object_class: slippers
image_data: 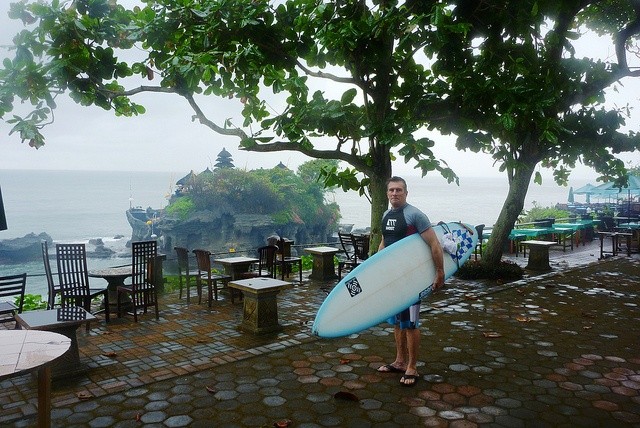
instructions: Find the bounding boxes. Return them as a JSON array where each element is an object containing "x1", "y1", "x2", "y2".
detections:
[
  {"x1": 376, "y1": 363, "x2": 406, "y2": 373},
  {"x1": 400, "y1": 370, "x2": 420, "y2": 387}
]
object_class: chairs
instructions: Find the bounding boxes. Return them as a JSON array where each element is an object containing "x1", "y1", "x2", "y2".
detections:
[
  {"x1": 337, "y1": 231, "x2": 369, "y2": 260},
  {"x1": 273, "y1": 242, "x2": 303, "y2": 282},
  {"x1": 41, "y1": 239, "x2": 87, "y2": 310},
  {"x1": 192, "y1": 247, "x2": 235, "y2": 308},
  {"x1": 244, "y1": 245, "x2": 279, "y2": 279},
  {"x1": 0, "y1": 272, "x2": 27, "y2": 330},
  {"x1": 174, "y1": 245, "x2": 207, "y2": 304},
  {"x1": 116, "y1": 240, "x2": 160, "y2": 324},
  {"x1": 468, "y1": 224, "x2": 486, "y2": 261},
  {"x1": 55, "y1": 242, "x2": 111, "y2": 332}
]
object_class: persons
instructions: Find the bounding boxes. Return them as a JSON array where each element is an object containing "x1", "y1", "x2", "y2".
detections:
[{"x1": 376, "y1": 176, "x2": 445, "y2": 388}]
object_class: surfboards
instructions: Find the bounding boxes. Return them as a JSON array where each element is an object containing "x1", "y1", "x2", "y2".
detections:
[{"x1": 310, "y1": 222, "x2": 479, "y2": 339}]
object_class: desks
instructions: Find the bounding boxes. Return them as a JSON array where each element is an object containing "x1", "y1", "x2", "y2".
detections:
[
  {"x1": 520, "y1": 239, "x2": 558, "y2": 271},
  {"x1": 482, "y1": 233, "x2": 527, "y2": 254},
  {"x1": 88, "y1": 268, "x2": 146, "y2": 310},
  {"x1": 213, "y1": 256, "x2": 260, "y2": 296},
  {"x1": 597, "y1": 231, "x2": 634, "y2": 261},
  {"x1": 227, "y1": 276, "x2": 294, "y2": 334},
  {"x1": 0, "y1": 327, "x2": 72, "y2": 428},
  {"x1": 556, "y1": 222, "x2": 586, "y2": 248},
  {"x1": 548, "y1": 227, "x2": 574, "y2": 253},
  {"x1": 14, "y1": 306, "x2": 97, "y2": 380},
  {"x1": 577, "y1": 219, "x2": 602, "y2": 242},
  {"x1": 569, "y1": 204, "x2": 639, "y2": 220},
  {"x1": 620, "y1": 222, "x2": 640, "y2": 248},
  {"x1": 512, "y1": 227, "x2": 550, "y2": 252},
  {"x1": 304, "y1": 244, "x2": 340, "y2": 281}
]
